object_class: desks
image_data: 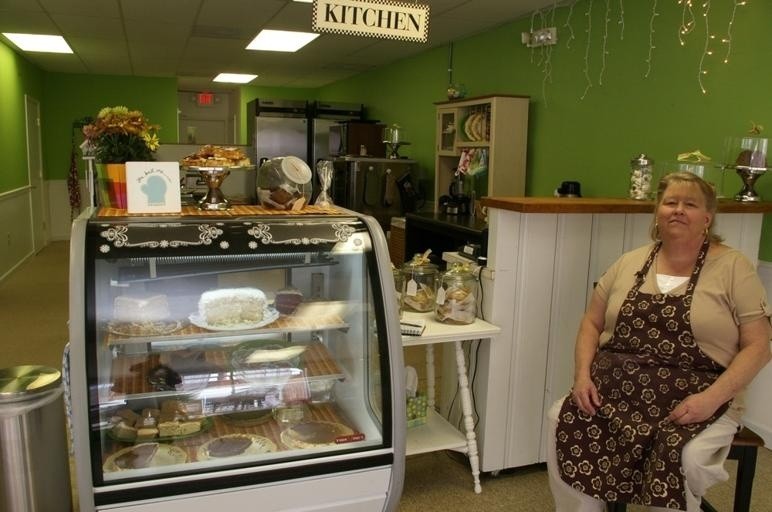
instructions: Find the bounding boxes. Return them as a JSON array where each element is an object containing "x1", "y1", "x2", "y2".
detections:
[{"x1": 371, "y1": 310, "x2": 502, "y2": 495}]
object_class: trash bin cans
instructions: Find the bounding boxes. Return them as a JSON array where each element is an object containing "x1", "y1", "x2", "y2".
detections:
[{"x1": 1, "y1": 365, "x2": 72, "y2": 511}]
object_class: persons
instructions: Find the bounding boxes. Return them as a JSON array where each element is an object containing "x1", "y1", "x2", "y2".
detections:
[{"x1": 544, "y1": 170, "x2": 772, "y2": 512}]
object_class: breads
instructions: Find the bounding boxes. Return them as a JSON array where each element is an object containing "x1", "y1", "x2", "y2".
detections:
[
  {"x1": 109, "y1": 400, "x2": 202, "y2": 440},
  {"x1": 181, "y1": 143, "x2": 250, "y2": 167}
]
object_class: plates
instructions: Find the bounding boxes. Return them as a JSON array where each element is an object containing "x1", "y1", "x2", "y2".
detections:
[{"x1": 465, "y1": 114, "x2": 489, "y2": 141}]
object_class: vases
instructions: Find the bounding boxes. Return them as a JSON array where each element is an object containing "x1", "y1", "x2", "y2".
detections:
[{"x1": 96, "y1": 165, "x2": 126, "y2": 209}]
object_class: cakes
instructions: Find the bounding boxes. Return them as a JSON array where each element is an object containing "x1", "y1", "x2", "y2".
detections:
[
  {"x1": 114, "y1": 444, "x2": 158, "y2": 468},
  {"x1": 293, "y1": 423, "x2": 342, "y2": 443},
  {"x1": 200, "y1": 288, "x2": 265, "y2": 323},
  {"x1": 207, "y1": 436, "x2": 252, "y2": 457},
  {"x1": 112, "y1": 292, "x2": 170, "y2": 320},
  {"x1": 275, "y1": 287, "x2": 303, "y2": 315}
]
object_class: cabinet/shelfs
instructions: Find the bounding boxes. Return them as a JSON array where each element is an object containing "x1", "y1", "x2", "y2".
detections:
[
  {"x1": 403, "y1": 211, "x2": 488, "y2": 273},
  {"x1": 70, "y1": 205, "x2": 406, "y2": 512},
  {"x1": 434, "y1": 94, "x2": 533, "y2": 215},
  {"x1": 330, "y1": 155, "x2": 417, "y2": 232},
  {"x1": 440, "y1": 193, "x2": 771, "y2": 477}
]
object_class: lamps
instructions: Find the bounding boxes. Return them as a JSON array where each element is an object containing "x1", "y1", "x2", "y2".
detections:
[{"x1": 520, "y1": 27, "x2": 557, "y2": 48}]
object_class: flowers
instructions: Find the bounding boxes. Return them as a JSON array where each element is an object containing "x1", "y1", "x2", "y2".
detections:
[{"x1": 81, "y1": 104, "x2": 162, "y2": 162}]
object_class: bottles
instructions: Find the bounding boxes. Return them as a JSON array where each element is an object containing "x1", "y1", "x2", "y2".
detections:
[
  {"x1": 625, "y1": 153, "x2": 657, "y2": 200},
  {"x1": 256, "y1": 156, "x2": 313, "y2": 210},
  {"x1": 447, "y1": 83, "x2": 465, "y2": 100},
  {"x1": 391, "y1": 257, "x2": 479, "y2": 325}
]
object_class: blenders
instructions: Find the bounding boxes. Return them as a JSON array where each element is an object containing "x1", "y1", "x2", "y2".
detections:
[{"x1": 447, "y1": 167, "x2": 472, "y2": 214}]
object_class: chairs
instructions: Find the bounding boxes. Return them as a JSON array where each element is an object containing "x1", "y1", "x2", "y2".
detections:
[{"x1": 593, "y1": 281, "x2": 765, "y2": 511}]
